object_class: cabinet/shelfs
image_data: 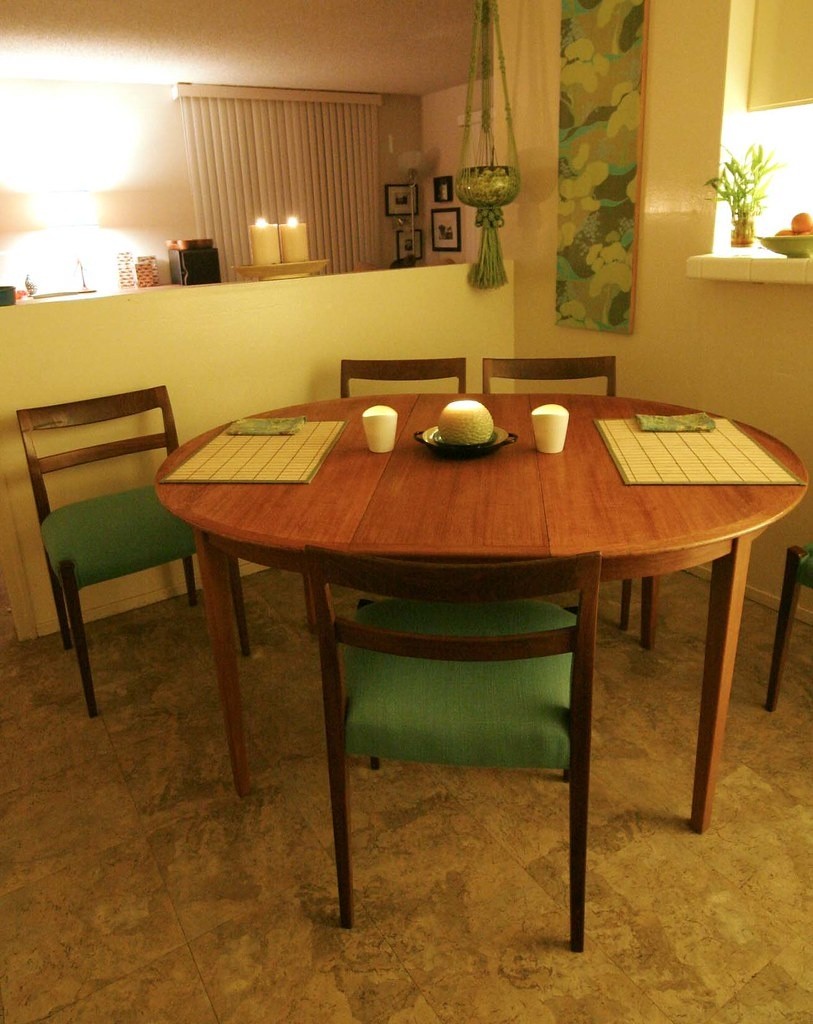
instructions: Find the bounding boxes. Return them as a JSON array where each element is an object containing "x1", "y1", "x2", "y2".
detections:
[{"x1": 169, "y1": 248, "x2": 221, "y2": 286}]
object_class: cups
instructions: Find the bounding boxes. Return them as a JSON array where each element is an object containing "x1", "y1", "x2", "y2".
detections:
[
  {"x1": 362, "y1": 405, "x2": 398, "y2": 453},
  {"x1": 531, "y1": 403, "x2": 570, "y2": 454}
]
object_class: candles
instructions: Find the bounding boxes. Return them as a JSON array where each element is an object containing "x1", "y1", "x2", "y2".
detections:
[
  {"x1": 249, "y1": 218, "x2": 280, "y2": 265},
  {"x1": 279, "y1": 217, "x2": 309, "y2": 263}
]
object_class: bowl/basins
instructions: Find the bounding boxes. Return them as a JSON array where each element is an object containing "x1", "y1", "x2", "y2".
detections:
[{"x1": 413, "y1": 425, "x2": 519, "y2": 458}]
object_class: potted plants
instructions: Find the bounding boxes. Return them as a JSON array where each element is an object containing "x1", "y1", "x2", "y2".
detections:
[{"x1": 703, "y1": 139, "x2": 784, "y2": 248}]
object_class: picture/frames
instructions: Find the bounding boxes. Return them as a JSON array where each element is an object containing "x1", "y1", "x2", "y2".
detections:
[
  {"x1": 396, "y1": 229, "x2": 423, "y2": 261},
  {"x1": 433, "y1": 176, "x2": 452, "y2": 202},
  {"x1": 384, "y1": 184, "x2": 418, "y2": 216},
  {"x1": 431, "y1": 207, "x2": 461, "y2": 251}
]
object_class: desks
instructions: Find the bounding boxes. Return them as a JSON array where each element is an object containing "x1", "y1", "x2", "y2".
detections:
[{"x1": 154, "y1": 393, "x2": 808, "y2": 834}]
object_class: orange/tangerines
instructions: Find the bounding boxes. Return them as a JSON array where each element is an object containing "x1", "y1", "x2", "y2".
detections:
[{"x1": 774, "y1": 212, "x2": 813, "y2": 236}]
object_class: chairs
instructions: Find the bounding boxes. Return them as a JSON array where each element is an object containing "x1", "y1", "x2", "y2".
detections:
[
  {"x1": 341, "y1": 357, "x2": 466, "y2": 397},
  {"x1": 483, "y1": 355, "x2": 632, "y2": 629},
  {"x1": 765, "y1": 541, "x2": 813, "y2": 713},
  {"x1": 304, "y1": 544, "x2": 603, "y2": 952},
  {"x1": 17, "y1": 385, "x2": 249, "y2": 718}
]
